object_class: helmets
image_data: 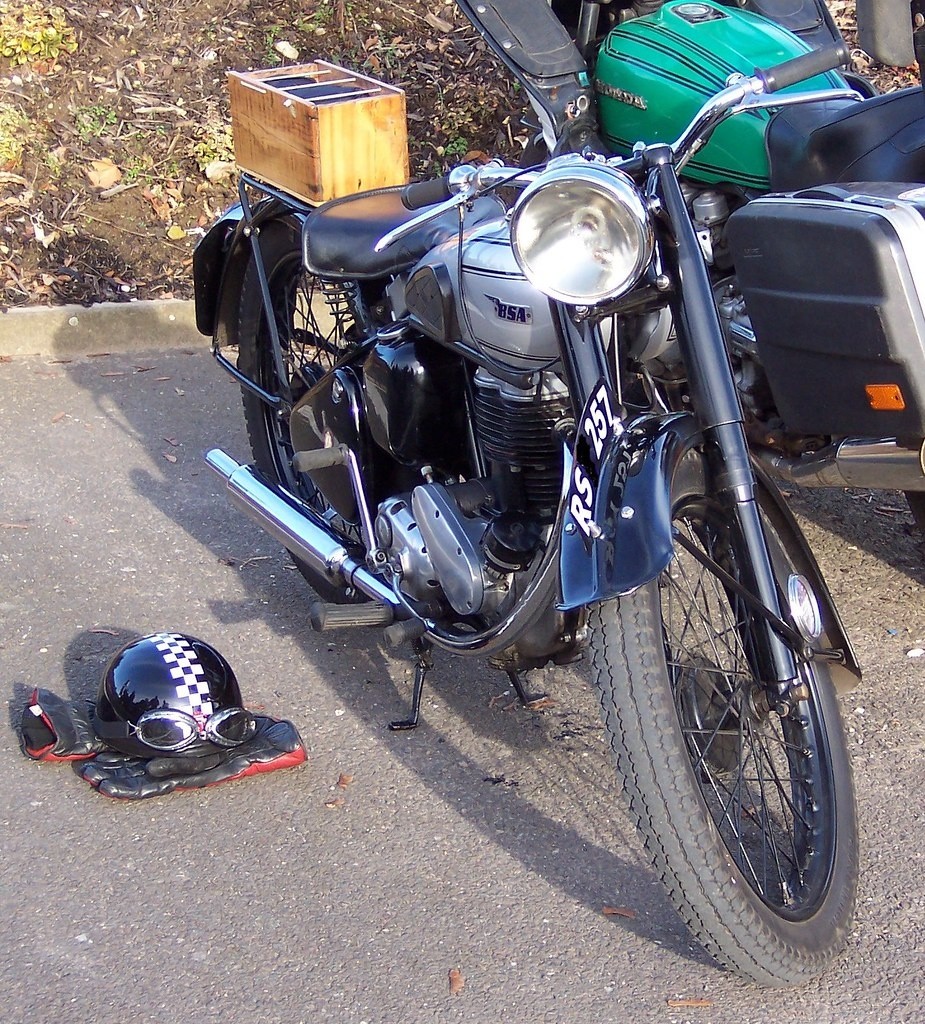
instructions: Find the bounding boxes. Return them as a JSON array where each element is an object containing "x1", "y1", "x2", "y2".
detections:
[{"x1": 91, "y1": 632, "x2": 243, "y2": 758}]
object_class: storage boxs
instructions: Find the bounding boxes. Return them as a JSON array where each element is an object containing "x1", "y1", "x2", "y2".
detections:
[{"x1": 227, "y1": 59, "x2": 410, "y2": 207}]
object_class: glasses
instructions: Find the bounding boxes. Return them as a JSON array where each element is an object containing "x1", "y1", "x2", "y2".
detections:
[{"x1": 93, "y1": 708, "x2": 257, "y2": 750}]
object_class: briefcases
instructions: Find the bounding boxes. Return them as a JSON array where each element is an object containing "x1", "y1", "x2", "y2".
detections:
[{"x1": 722, "y1": 182, "x2": 925, "y2": 439}]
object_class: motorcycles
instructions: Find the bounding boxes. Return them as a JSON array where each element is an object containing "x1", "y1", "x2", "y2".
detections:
[
  {"x1": 456, "y1": 0, "x2": 925, "y2": 542},
  {"x1": 192, "y1": 37, "x2": 866, "y2": 993}
]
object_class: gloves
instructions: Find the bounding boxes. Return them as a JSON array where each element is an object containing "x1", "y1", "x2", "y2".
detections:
[
  {"x1": 21, "y1": 687, "x2": 124, "y2": 761},
  {"x1": 80, "y1": 713, "x2": 307, "y2": 799}
]
